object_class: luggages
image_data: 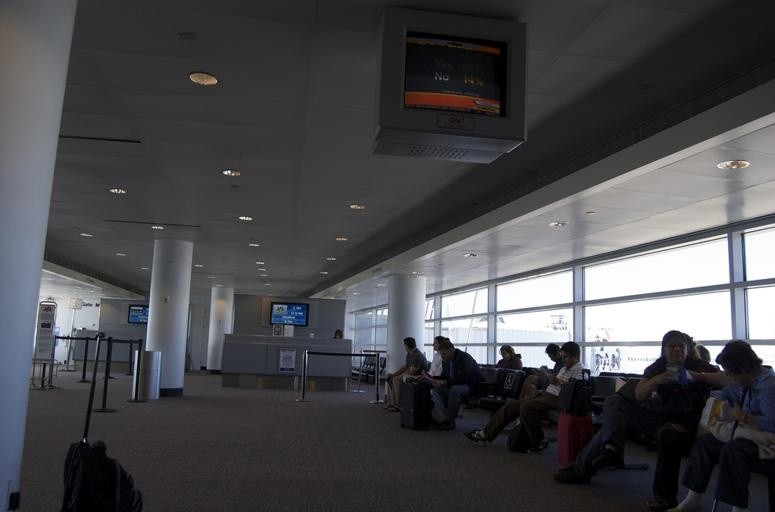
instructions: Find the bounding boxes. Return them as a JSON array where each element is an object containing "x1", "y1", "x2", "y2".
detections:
[
  {"x1": 58, "y1": 441, "x2": 144, "y2": 512},
  {"x1": 397, "y1": 376, "x2": 433, "y2": 432},
  {"x1": 555, "y1": 410, "x2": 594, "y2": 466}
]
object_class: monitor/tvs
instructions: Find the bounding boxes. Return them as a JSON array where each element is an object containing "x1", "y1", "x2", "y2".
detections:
[
  {"x1": 370, "y1": 8, "x2": 527, "y2": 164},
  {"x1": 270, "y1": 301, "x2": 309, "y2": 327},
  {"x1": 128, "y1": 304, "x2": 149, "y2": 324}
]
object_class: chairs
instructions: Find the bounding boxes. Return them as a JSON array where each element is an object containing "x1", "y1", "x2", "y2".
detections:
[
  {"x1": 463, "y1": 364, "x2": 535, "y2": 414},
  {"x1": 352, "y1": 355, "x2": 386, "y2": 383},
  {"x1": 589, "y1": 372, "x2": 775, "y2": 512}
]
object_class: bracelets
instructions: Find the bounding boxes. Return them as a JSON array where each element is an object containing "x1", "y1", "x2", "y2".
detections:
[
  {"x1": 700, "y1": 372, "x2": 706, "y2": 381},
  {"x1": 649, "y1": 378, "x2": 657, "y2": 386},
  {"x1": 742, "y1": 412, "x2": 750, "y2": 424},
  {"x1": 559, "y1": 380, "x2": 564, "y2": 386}
]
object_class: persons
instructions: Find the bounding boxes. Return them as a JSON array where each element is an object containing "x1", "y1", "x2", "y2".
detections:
[
  {"x1": 540, "y1": 342, "x2": 566, "y2": 375},
  {"x1": 460, "y1": 342, "x2": 591, "y2": 455},
  {"x1": 429, "y1": 341, "x2": 490, "y2": 430},
  {"x1": 493, "y1": 344, "x2": 524, "y2": 372},
  {"x1": 603, "y1": 329, "x2": 732, "y2": 511},
  {"x1": 420, "y1": 336, "x2": 448, "y2": 378},
  {"x1": 384, "y1": 336, "x2": 428, "y2": 412},
  {"x1": 332, "y1": 329, "x2": 343, "y2": 340},
  {"x1": 553, "y1": 333, "x2": 699, "y2": 484},
  {"x1": 666, "y1": 341, "x2": 775, "y2": 512}
]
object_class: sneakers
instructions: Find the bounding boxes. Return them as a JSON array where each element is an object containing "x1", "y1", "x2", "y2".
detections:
[
  {"x1": 435, "y1": 419, "x2": 457, "y2": 432},
  {"x1": 461, "y1": 430, "x2": 493, "y2": 445},
  {"x1": 644, "y1": 497, "x2": 701, "y2": 511},
  {"x1": 552, "y1": 464, "x2": 579, "y2": 484},
  {"x1": 526, "y1": 438, "x2": 551, "y2": 455},
  {"x1": 384, "y1": 403, "x2": 400, "y2": 414}
]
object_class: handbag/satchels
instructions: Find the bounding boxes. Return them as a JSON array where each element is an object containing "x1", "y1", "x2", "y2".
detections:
[{"x1": 559, "y1": 376, "x2": 591, "y2": 416}]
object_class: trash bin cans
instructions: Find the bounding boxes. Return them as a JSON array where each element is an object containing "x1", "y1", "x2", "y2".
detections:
[{"x1": 132, "y1": 350, "x2": 161, "y2": 400}]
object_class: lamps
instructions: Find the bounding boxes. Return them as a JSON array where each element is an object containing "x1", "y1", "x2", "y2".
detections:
[{"x1": 59, "y1": 297, "x2": 84, "y2": 371}]
